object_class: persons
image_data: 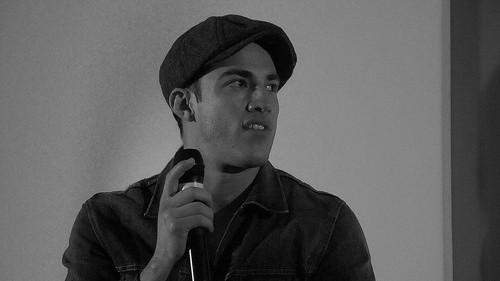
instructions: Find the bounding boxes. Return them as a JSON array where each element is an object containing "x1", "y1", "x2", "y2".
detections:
[{"x1": 61, "y1": 14, "x2": 377, "y2": 281}]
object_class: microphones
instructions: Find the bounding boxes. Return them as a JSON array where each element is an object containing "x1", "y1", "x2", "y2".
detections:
[{"x1": 173, "y1": 149, "x2": 211, "y2": 281}]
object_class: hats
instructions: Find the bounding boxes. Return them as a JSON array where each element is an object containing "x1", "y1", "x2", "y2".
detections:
[{"x1": 159, "y1": 12, "x2": 297, "y2": 107}]
